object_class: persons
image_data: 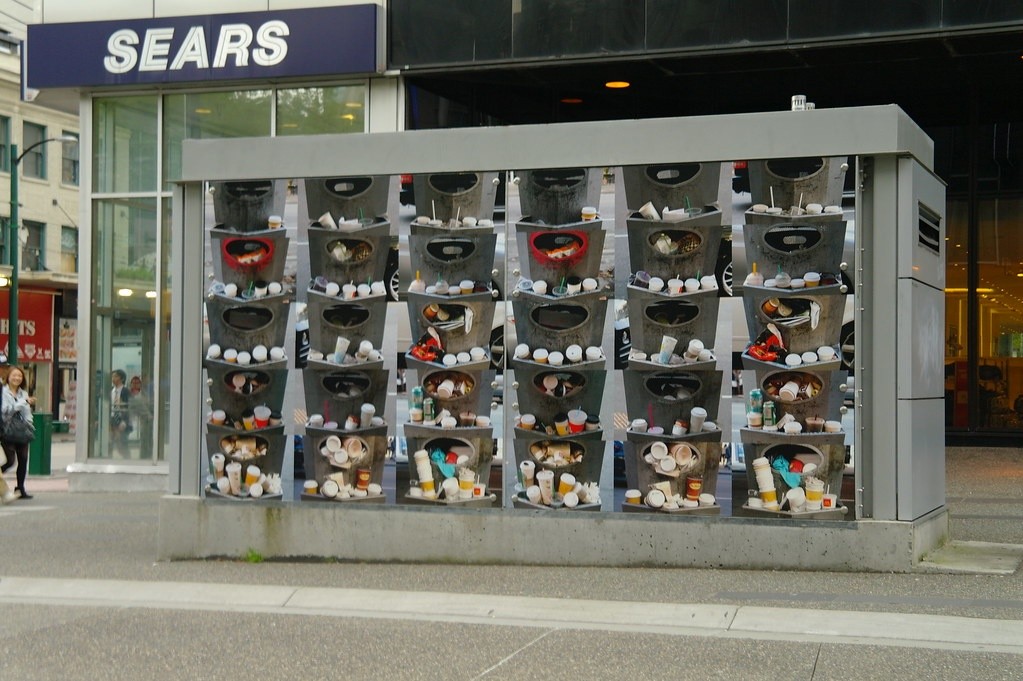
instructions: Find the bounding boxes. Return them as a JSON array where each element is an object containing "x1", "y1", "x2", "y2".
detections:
[
  {"x1": 0, "y1": 353, "x2": 38, "y2": 504},
  {"x1": 108, "y1": 369, "x2": 142, "y2": 461}
]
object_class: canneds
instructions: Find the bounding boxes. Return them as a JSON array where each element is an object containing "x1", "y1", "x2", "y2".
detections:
[
  {"x1": 412, "y1": 386, "x2": 423, "y2": 410},
  {"x1": 763, "y1": 402, "x2": 776, "y2": 426},
  {"x1": 750, "y1": 389, "x2": 763, "y2": 413},
  {"x1": 424, "y1": 398, "x2": 434, "y2": 420}
]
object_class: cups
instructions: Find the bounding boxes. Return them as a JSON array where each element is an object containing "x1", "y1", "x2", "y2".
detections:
[
  {"x1": 625, "y1": 442, "x2": 715, "y2": 509},
  {"x1": 411, "y1": 302, "x2": 485, "y2": 366},
  {"x1": 520, "y1": 409, "x2": 599, "y2": 436},
  {"x1": 409, "y1": 445, "x2": 485, "y2": 499},
  {"x1": 760, "y1": 296, "x2": 835, "y2": 366},
  {"x1": 304, "y1": 470, "x2": 382, "y2": 498},
  {"x1": 633, "y1": 271, "x2": 715, "y2": 294},
  {"x1": 632, "y1": 407, "x2": 715, "y2": 435},
  {"x1": 318, "y1": 211, "x2": 372, "y2": 231},
  {"x1": 515, "y1": 344, "x2": 601, "y2": 366},
  {"x1": 581, "y1": 206, "x2": 596, "y2": 222},
  {"x1": 747, "y1": 381, "x2": 841, "y2": 433},
  {"x1": 408, "y1": 280, "x2": 474, "y2": 295},
  {"x1": 746, "y1": 272, "x2": 835, "y2": 289},
  {"x1": 417, "y1": 216, "x2": 491, "y2": 227},
  {"x1": 634, "y1": 335, "x2": 710, "y2": 365},
  {"x1": 313, "y1": 276, "x2": 386, "y2": 298},
  {"x1": 520, "y1": 441, "x2": 589, "y2": 506},
  {"x1": 685, "y1": 208, "x2": 702, "y2": 216},
  {"x1": 409, "y1": 378, "x2": 490, "y2": 428},
  {"x1": 639, "y1": 201, "x2": 661, "y2": 221},
  {"x1": 233, "y1": 374, "x2": 245, "y2": 388},
  {"x1": 209, "y1": 405, "x2": 282, "y2": 430},
  {"x1": 753, "y1": 203, "x2": 840, "y2": 215},
  {"x1": 311, "y1": 336, "x2": 378, "y2": 364},
  {"x1": 212, "y1": 437, "x2": 270, "y2": 497},
  {"x1": 208, "y1": 344, "x2": 284, "y2": 366},
  {"x1": 269, "y1": 215, "x2": 280, "y2": 230},
  {"x1": 310, "y1": 404, "x2": 383, "y2": 431},
  {"x1": 520, "y1": 276, "x2": 597, "y2": 296},
  {"x1": 212, "y1": 282, "x2": 281, "y2": 298},
  {"x1": 748, "y1": 457, "x2": 837, "y2": 512}
]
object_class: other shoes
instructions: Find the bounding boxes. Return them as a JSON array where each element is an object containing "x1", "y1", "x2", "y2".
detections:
[{"x1": 14, "y1": 486, "x2": 32, "y2": 499}]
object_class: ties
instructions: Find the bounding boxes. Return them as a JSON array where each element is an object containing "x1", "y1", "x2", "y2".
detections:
[{"x1": 114, "y1": 392, "x2": 120, "y2": 425}]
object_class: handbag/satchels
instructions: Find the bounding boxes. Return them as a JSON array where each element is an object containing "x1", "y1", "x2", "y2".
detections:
[{"x1": 2, "y1": 411, "x2": 35, "y2": 443}]
